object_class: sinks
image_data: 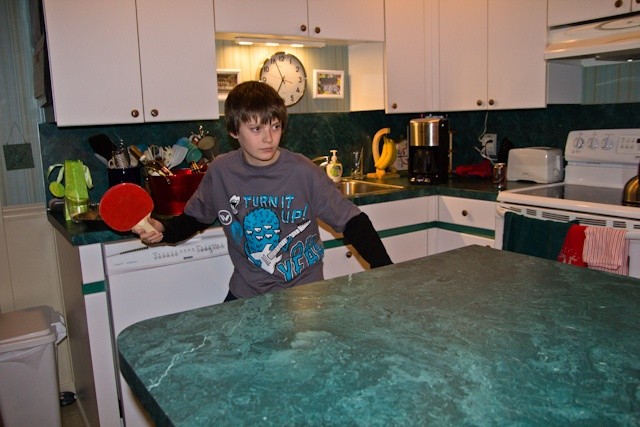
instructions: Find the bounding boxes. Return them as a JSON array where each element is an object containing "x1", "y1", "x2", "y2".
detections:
[{"x1": 336, "y1": 179, "x2": 407, "y2": 198}]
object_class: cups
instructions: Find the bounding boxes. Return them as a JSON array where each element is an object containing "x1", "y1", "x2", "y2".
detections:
[
  {"x1": 350, "y1": 151, "x2": 364, "y2": 180},
  {"x1": 175, "y1": 136, "x2": 203, "y2": 164},
  {"x1": 64, "y1": 187, "x2": 90, "y2": 223},
  {"x1": 127, "y1": 145, "x2": 143, "y2": 167},
  {"x1": 197, "y1": 134, "x2": 219, "y2": 159},
  {"x1": 108, "y1": 149, "x2": 139, "y2": 169}
]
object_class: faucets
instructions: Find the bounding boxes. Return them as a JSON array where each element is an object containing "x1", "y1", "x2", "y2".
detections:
[{"x1": 312, "y1": 156, "x2": 330, "y2": 168}]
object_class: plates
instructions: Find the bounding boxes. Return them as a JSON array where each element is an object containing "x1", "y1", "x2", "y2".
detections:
[{"x1": 452, "y1": 173, "x2": 482, "y2": 186}]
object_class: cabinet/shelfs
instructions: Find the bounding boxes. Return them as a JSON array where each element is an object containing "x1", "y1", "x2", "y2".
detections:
[
  {"x1": 384, "y1": 1, "x2": 549, "y2": 114},
  {"x1": 40, "y1": 1, "x2": 221, "y2": 128},
  {"x1": 212, "y1": 0, "x2": 385, "y2": 43},
  {"x1": 547, "y1": 1, "x2": 640, "y2": 30},
  {"x1": 318, "y1": 195, "x2": 497, "y2": 281}
]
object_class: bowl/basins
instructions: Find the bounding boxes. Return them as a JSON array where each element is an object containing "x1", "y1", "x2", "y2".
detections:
[
  {"x1": 73, "y1": 205, "x2": 109, "y2": 226},
  {"x1": 141, "y1": 158, "x2": 219, "y2": 215}
]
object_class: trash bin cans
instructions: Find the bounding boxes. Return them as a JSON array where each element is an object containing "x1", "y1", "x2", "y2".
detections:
[{"x1": 1, "y1": 306, "x2": 68, "y2": 427}]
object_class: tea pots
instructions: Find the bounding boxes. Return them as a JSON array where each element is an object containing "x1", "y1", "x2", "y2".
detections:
[{"x1": 621, "y1": 162, "x2": 640, "y2": 208}]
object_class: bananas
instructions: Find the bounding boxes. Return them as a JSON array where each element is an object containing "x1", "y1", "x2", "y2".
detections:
[{"x1": 374, "y1": 137, "x2": 396, "y2": 169}]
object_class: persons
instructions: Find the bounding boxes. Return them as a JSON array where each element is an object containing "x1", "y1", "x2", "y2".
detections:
[{"x1": 129, "y1": 79, "x2": 395, "y2": 307}]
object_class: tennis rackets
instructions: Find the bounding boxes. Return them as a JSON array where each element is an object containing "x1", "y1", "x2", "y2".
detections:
[{"x1": 99, "y1": 183, "x2": 159, "y2": 235}]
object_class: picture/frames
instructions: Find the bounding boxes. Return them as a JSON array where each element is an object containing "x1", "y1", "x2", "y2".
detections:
[
  {"x1": 312, "y1": 68, "x2": 345, "y2": 100},
  {"x1": 215, "y1": 68, "x2": 243, "y2": 101}
]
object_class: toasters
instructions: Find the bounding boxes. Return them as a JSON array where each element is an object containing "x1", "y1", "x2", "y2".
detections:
[{"x1": 507, "y1": 147, "x2": 564, "y2": 184}]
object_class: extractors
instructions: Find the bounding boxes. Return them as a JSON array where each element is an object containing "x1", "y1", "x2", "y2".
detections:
[{"x1": 543, "y1": 14, "x2": 640, "y2": 68}]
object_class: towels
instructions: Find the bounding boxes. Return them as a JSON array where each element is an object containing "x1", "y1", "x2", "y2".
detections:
[
  {"x1": 502, "y1": 210, "x2": 580, "y2": 260},
  {"x1": 583, "y1": 225, "x2": 629, "y2": 278},
  {"x1": 557, "y1": 224, "x2": 587, "y2": 267}
]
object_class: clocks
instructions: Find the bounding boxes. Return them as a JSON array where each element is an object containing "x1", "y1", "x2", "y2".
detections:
[{"x1": 258, "y1": 51, "x2": 308, "y2": 109}]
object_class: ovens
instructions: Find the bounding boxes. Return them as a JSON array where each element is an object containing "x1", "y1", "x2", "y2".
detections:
[{"x1": 494, "y1": 201, "x2": 640, "y2": 280}]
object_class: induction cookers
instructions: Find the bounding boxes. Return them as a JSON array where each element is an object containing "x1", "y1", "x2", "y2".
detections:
[{"x1": 495, "y1": 164, "x2": 640, "y2": 220}]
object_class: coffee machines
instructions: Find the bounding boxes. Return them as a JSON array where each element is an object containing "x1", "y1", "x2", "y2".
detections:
[{"x1": 406, "y1": 116, "x2": 449, "y2": 186}]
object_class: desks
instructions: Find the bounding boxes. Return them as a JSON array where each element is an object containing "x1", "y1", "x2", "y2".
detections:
[{"x1": 115, "y1": 244, "x2": 640, "y2": 426}]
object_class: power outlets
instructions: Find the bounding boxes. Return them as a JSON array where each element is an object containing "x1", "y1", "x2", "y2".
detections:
[{"x1": 480, "y1": 134, "x2": 497, "y2": 156}]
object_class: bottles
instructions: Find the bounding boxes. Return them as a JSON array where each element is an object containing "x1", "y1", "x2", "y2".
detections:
[{"x1": 49, "y1": 158, "x2": 94, "y2": 221}]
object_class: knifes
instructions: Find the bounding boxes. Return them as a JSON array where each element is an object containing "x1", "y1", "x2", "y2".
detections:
[{"x1": 94, "y1": 153, "x2": 108, "y2": 167}]
